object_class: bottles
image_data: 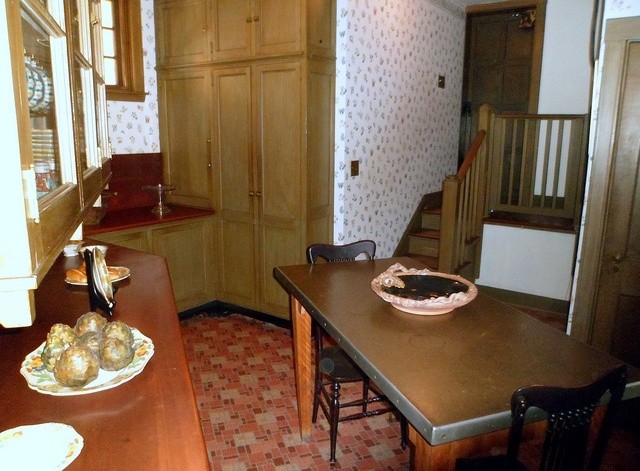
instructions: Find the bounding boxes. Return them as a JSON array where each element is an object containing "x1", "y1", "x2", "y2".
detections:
[{"x1": 47, "y1": 161, "x2": 59, "y2": 186}]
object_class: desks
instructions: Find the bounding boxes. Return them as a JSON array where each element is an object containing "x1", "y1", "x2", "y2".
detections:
[{"x1": 0, "y1": 238, "x2": 211, "y2": 469}]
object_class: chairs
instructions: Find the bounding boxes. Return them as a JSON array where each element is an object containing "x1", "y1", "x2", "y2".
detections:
[
  {"x1": 306, "y1": 241, "x2": 408, "y2": 465},
  {"x1": 456, "y1": 364, "x2": 627, "y2": 471}
]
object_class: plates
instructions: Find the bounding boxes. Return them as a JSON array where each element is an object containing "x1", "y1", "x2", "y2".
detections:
[
  {"x1": 65, "y1": 266, "x2": 131, "y2": 286},
  {"x1": 19, "y1": 325, "x2": 156, "y2": 397},
  {"x1": 0, "y1": 421, "x2": 84, "y2": 471},
  {"x1": 371, "y1": 266, "x2": 478, "y2": 315},
  {"x1": 91, "y1": 245, "x2": 114, "y2": 305},
  {"x1": 24, "y1": 64, "x2": 56, "y2": 116},
  {"x1": 31, "y1": 128, "x2": 60, "y2": 163}
]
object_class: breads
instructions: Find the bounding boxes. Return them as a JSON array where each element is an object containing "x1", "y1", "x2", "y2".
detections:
[
  {"x1": 41, "y1": 311, "x2": 136, "y2": 387},
  {"x1": 66, "y1": 262, "x2": 131, "y2": 283}
]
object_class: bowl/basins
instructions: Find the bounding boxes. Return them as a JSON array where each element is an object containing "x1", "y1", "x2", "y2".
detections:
[
  {"x1": 63, "y1": 240, "x2": 86, "y2": 257},
  {"x1": 74, "y1": 245, "x2": 109, "y2": 260}
]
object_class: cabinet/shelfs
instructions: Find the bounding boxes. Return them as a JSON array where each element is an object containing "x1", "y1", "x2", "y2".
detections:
[
  {"x1": 214, "y1": 208, "x2": 334, "y2": 331},
  {"x1": 6, "y1": 0, "x2": 111, "y2": 287},
  {"x1": 216, "y1": 62, "x2": 334, "y2": 225},
  {"x1": 156, "y1": 68, "x2": 215, "y2": 213},
  {"x1": 155, "y1": 1, "x2": 210, "y2": 68},
  {"x1": 87, "y1": 214, "x2": 213, "y2": 322},
  {"x1": 211, "y1": 0, "x2": 335, "y2": 62}
]
